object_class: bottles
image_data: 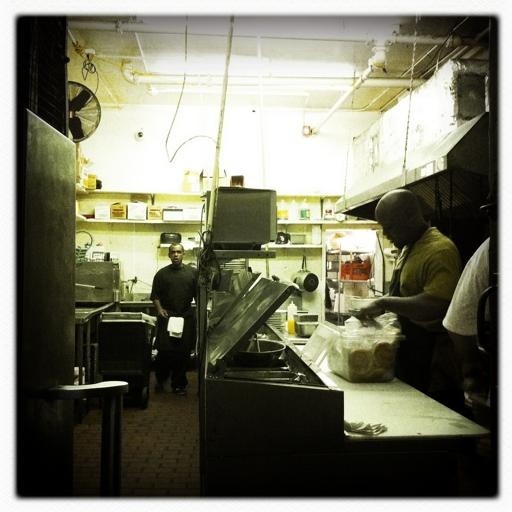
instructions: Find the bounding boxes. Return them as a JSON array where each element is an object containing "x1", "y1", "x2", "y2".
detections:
[
  {"x1": 287, "y1": 299, "x2": 298, "y2": 335},
  {"x1": 276, "y1": 197, "x2": 312, "y2": 220},
  {"x1": 324, "y1": 198, "x2": 335, "y2": 220}
]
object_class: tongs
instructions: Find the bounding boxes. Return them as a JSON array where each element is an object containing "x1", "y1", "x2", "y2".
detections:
[{"x1": 248, "y1": 333, "x2": 261, "y2": 352}]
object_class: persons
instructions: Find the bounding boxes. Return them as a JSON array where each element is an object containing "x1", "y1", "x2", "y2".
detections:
[
  {"x1": 149, "y1": 241, "x2": 199, "y2": 396},
  {"x1": 429, "y1": 191, "x2": 497, "y2": 451},
  {"x1": 346, "y1": 188, "x2": 466, "y2": 419}
]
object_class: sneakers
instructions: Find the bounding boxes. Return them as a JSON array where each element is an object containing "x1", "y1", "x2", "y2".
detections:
[
  {"x1": 156, "y1": 381, "x2": 163, "y2": 392},
  {"x1": 173, "y1": 387, "x2": 186, "y2": 395}
]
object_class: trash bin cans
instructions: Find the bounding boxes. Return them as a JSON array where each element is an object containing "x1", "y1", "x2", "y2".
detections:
[{"x1": 99, "y1": 312, "x2": 156, "y2": 409}]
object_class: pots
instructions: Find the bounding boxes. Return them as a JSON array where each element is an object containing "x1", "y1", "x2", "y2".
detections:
[{"x1": 292, "y1": 252, "x2": 320, "y2": 294}]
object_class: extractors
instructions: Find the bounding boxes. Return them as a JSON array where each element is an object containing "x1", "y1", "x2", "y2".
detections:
[{"x1": 331, "y1": 109, "x2": 497, "y2": 229}]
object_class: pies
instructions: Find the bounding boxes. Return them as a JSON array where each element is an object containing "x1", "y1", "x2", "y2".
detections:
[{"x1": 328, "y1": 337, "x2": 397, "y2": 383}]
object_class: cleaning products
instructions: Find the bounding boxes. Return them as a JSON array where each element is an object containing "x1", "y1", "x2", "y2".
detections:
[
  {"x1": 322, "y1": 199, "x2": 333, "y2": 220},
  {"x1": 288, "y1": 200, "x2": 299, "y2": 220},
  {"x1": 276, "y1": 199, "x2": 288, "y2": 221},
  {"x1": 298, "y1": 197, "x2": 311, "y2": 220}
]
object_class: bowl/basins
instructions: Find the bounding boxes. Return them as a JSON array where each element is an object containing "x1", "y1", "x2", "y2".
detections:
[{"x1": 235, "y1": 338, "x2": 287, "y2": 366}]
